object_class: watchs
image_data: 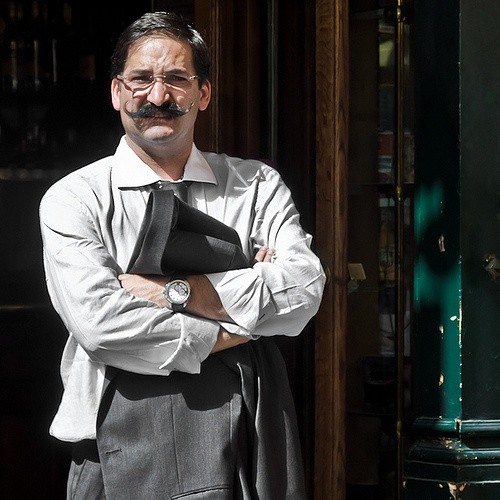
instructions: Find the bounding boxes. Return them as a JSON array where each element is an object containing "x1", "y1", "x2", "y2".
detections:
[{"x1": 161, "y1": 276, "x2": 192, "y2": 310}]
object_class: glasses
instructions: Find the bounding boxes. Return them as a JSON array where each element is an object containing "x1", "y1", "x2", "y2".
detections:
[{"x1": 117, "y1": 73, "x2": 199, "y2": 90}]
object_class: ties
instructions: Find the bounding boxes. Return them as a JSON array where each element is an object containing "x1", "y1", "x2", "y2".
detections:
[{"x1": 152, "y1": 181, "x2": 193, "y2": 205}]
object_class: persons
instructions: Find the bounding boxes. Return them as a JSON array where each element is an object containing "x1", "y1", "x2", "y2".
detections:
[{"x1": 39, "y1": 11, "x2": 327, "y2": 500}]
